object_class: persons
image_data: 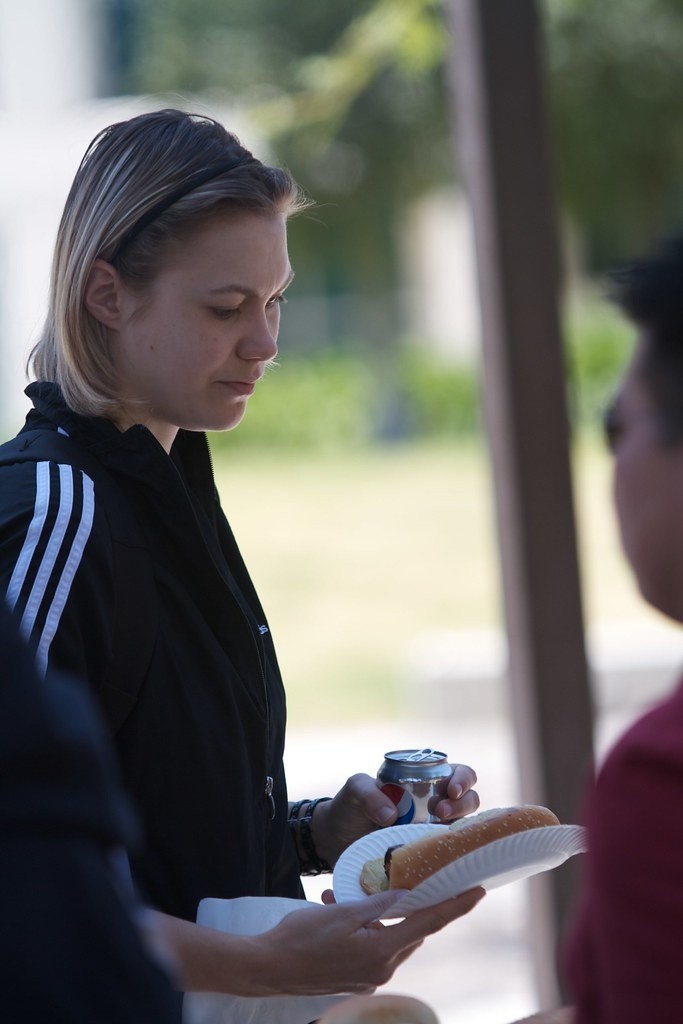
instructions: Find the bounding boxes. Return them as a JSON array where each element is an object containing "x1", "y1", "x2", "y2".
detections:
[
  {"x1": 0, "y1": 110, "x2": 486, "y2": 1024},
  {"x1": 510, "y1": 237, "x2": 683, "y2": 1023}
]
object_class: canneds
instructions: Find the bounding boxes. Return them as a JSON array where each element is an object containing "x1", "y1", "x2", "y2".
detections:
[{"x1": 375, "y1": 749, "x2": 453, "y2": 826}]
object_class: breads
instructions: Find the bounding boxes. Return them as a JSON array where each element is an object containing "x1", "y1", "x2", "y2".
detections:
[{"x1": 314, "y1": 994, "x2": 438, "y2": 1024}]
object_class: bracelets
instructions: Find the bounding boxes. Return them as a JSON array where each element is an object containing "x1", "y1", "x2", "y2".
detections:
[{"x1": 287, "y1": 797, "x2": 334, "y2": 879}]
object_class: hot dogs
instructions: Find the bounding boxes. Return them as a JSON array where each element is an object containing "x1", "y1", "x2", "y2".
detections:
[{"x1": 360, "y1": 803, "x2": 563, "y2": 895}]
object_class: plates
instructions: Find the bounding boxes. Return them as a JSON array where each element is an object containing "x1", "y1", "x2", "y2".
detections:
[{"x1": 332, "y1": 822, "x2": 591, "y2": 925}]
